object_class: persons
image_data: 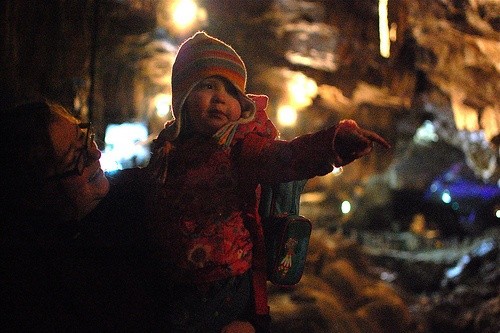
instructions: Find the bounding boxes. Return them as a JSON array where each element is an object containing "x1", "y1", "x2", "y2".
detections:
[
  {"x1": 103, "y1": 32, "x2": 389, "y2": 333},
  {"x1": 0, "y1": 99, "x2": 273, "y2": 333}
]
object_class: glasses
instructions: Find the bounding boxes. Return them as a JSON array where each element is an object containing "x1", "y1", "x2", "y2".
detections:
[{"x1": 36, "y1": 120, "x2": 95, "y2": 183}]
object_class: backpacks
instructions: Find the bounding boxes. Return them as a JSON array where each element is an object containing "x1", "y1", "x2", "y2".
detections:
[{"x1": 233, "y1": 131, "x2": 313, "y2": 290}]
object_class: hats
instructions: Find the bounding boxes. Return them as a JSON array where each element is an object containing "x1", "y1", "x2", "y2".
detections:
[{"x1": 156, "y1": 30, "x2": 256, "y2": 157}]
